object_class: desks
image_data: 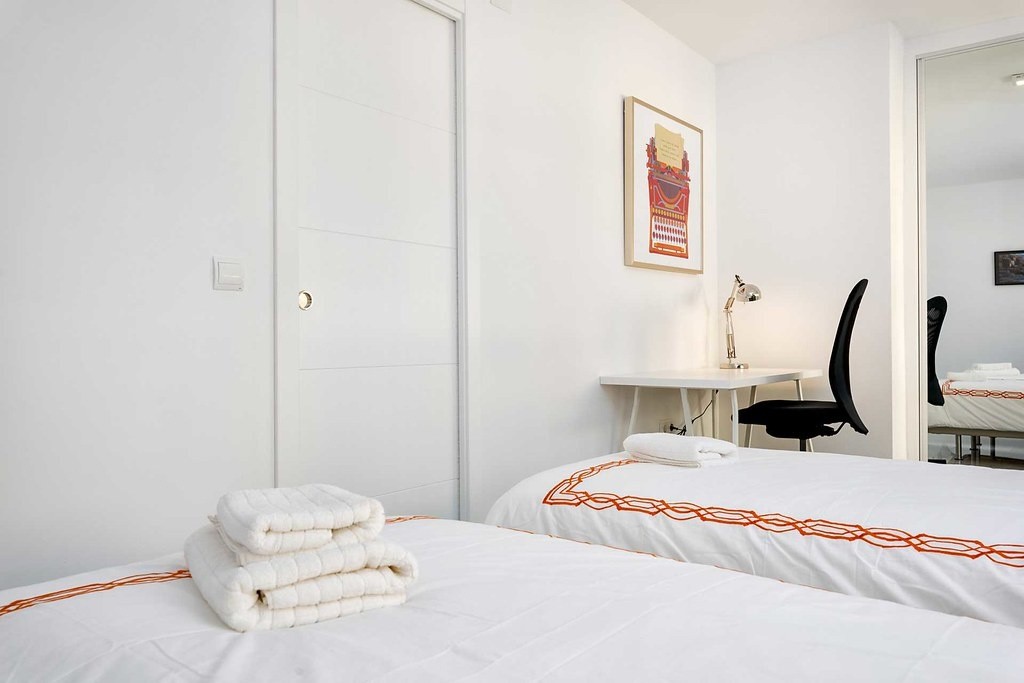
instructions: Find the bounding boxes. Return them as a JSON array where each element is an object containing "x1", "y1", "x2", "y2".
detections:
[{"x1": 600, "y1": 368, "x2": 822, "y2": 448}]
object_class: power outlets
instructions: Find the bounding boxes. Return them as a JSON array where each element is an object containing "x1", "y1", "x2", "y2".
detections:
[{"x1": 658, "y1": 418, "x2": 673, "y2": 432}]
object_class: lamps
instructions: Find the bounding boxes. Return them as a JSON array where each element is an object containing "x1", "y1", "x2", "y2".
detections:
[
  {"x1": 1011, "y1": 73, "x2": 1024, "y2": 86},
  {"x1": 719, "y1": 275, "x2": 761, "y2": 368}
]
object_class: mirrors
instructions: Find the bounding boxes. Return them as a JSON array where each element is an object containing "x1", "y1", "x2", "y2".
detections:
[{"x1": 917, "y1": 33, "x2": 1024, "y2": 470}]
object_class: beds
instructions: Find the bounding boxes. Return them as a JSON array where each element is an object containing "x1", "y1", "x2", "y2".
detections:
[
  {"x1": 484, "y1": 451, "x2": 1024, "y2": 629},
  {"x1": 927, "y1": 380, "x2": 1024, "y2": 470},
  {"x1": 0, "y1": 511, "x2": 1024, "y2": 683}
]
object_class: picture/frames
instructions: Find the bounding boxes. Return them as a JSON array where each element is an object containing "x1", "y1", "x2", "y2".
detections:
[
  {"x1": 993, "y1": 250, "x2": 1024, "y2": 285},
  {"x1": 624, "y1": 95, "x2": 704, "y2": 274}
]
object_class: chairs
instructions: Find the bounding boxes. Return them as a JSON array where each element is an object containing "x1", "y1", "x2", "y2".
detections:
[
  {"x1": 927, "y1": 295, "x2": 948, "y2": 464},
  {"x1": 730, "y1": 278, "x2": 869, "y2": 451}
]
